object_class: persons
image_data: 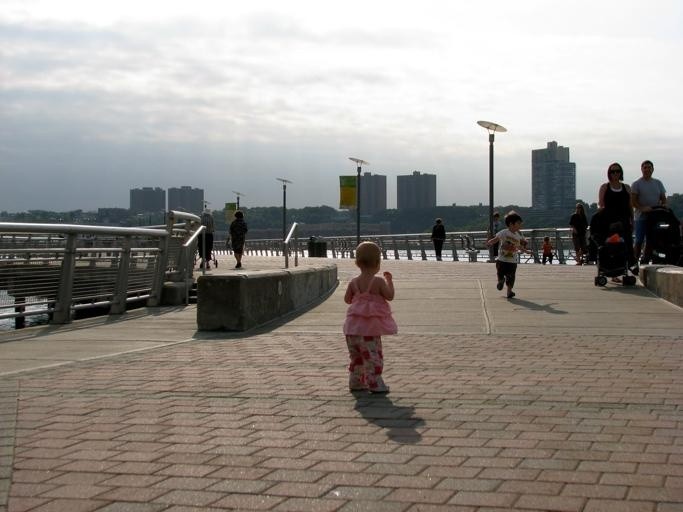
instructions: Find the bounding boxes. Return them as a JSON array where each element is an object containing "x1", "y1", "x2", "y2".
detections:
[
  {"x1": 487, "y1": 211, "x2": 529, "y2": 299},
  {"x1": 430, "y1": 218, "x2": 445, "y2": 261},
  {"x1": 569, "y1": 202, "x2": 589, "y2": 266},
  {"x1": 541, "y1": 236, "x2": 554, "y2": 265},
  {"x1": 487, "y1": 211, "x2": 503, "y2": 258},
  {"x1": 604, "y1": 221, "x2": 625, "y2": 243},
  {"x1": 632, "y1": 160, "x2": 667, "y2": 263},
  {"x1": 342, "y1": 241, "x2": 398, "y2": 393},
  {"x1": 598, "y1": 162, "x2": 637, "y2": 283},
  {"x1": 197, "y1": 208, "x2": 214, "y2": 268},
  {"x1": 228, "y1": 209, "x2": 248, "y2": 269}
]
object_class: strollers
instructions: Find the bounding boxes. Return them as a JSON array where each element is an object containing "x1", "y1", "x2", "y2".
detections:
[
  {"x1": 639, "y1": 204, "x2": 682, "y2": 267},
  {"x1": 587, "y1": 209, "x2": 637, "y2": 288},
  {"x1": 191, "y1": 232, "x2": 219, "y2": 267}
]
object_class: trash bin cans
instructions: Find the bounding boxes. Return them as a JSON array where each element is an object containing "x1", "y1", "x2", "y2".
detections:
[{"x1": 307, "y1": 236, "x2": 327, "y2": 257}]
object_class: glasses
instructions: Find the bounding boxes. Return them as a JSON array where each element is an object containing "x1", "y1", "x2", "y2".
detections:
[{"x1": 611, "y1": 169, "x2": 620, "y2": 174}]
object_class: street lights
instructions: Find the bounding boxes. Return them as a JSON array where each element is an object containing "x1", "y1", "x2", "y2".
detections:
[
  {"x1": 114, "y1": 206, "x2": 190, "y2": 228},
  {"x1": 348, "y1": 155, "x2": 370, "y2": 251},
  {"x1": 231, "y1": 189, "x2": 246, "y2": 211},
  {"x1": 199, "y1": 198, "x2": 212, "y2": 211},
  {"x1": 476, "y1": 119, "x2": 507, "y2": 265},
  {"x1": 276, "y1": 174, "x2": 293, "y2": 256}
]
